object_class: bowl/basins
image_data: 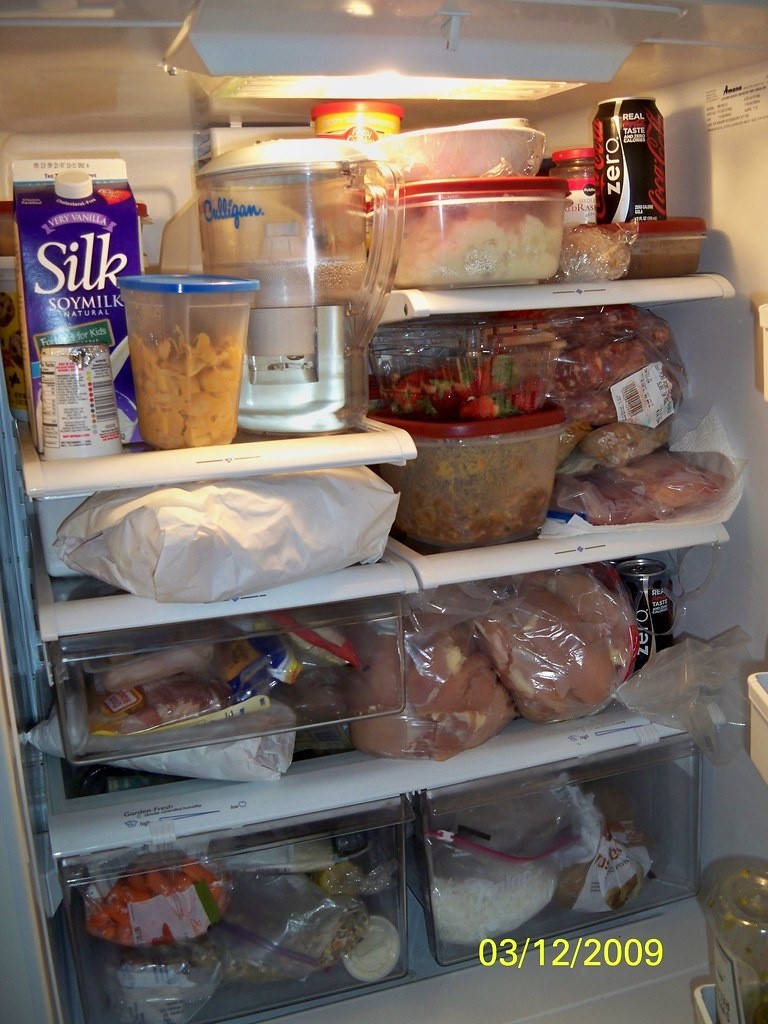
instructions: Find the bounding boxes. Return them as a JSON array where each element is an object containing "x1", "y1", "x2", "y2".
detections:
[
  {"x1": 390, "y1": 116, "x2": 548, "y2": 185},
  {"x1": 571, "y1": 216, "x2": 708, "y2": 280},
  {"x1": 365, "y1": 398, "x2": 566, "y2": 550},
  {"x1": 368, "y1": 312, "x2": 566, "y2": 421},
  {"x1": 363, "y1": 176, "x2": 573, "y2": 289}
]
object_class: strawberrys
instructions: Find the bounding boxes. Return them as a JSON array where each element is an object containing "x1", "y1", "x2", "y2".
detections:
[{"x1": 384, "y1": 347, "x2": 546, "y2": 420}]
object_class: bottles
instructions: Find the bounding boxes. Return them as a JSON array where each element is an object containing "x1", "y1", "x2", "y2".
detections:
[
  {"x1": 706, "y1": 868, "x2": 768, "y2": 1024},
  {"x1": 116, "y1": 272, "x2": 261, "y2": 449},
  {"x1": 549, "y1": 147, "x2": 596, "y2": 225}
]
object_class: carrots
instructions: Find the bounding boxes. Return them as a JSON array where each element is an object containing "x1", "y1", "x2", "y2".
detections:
[{"x1": 86, "y1": 852, "x2": 226, "y2": 946}]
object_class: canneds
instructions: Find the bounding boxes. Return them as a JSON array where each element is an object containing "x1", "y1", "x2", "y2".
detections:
[
  {"x1": 614, "y1": 559, "x2": 674, "y2": 669},
  {"x1": 591, "y1": 96, "x2": 667, "y2": 225},
  {"x1": 550, "y1": 148, "x2": 598, "y2": 226}
]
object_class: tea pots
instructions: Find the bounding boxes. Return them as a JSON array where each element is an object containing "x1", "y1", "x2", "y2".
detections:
[{"x1": 193, "y1": 136, "x2": 407, "y2": 433}]
